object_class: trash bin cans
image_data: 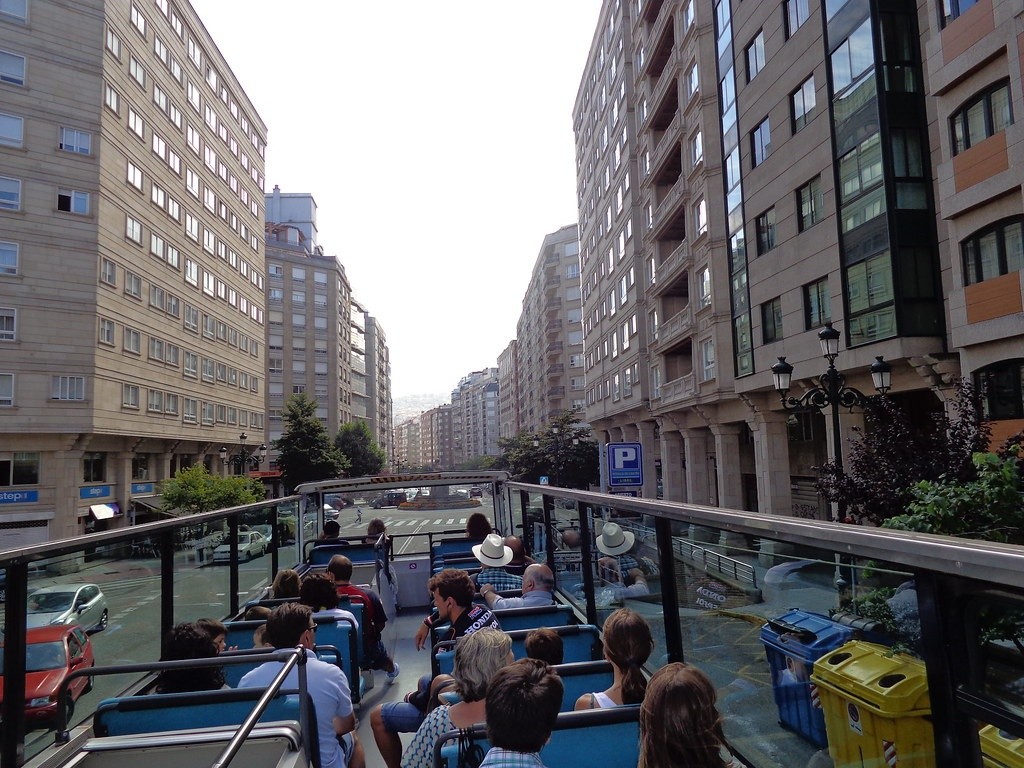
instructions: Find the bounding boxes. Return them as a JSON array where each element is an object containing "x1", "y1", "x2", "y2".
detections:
[
  {"x1": 810, "y1": 640, "x2": 936, "y2": 768},
  {"x1": 758, "y1": 609, "x2": 863, "y2": 747},
  {"x1": 979, "y1": 725, "x2": 1024, "y2": 768}
]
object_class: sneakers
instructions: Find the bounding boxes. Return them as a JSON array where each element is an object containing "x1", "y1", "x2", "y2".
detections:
[{"x1": 384, "y1": 663, "x2": 399, "y2": 682}]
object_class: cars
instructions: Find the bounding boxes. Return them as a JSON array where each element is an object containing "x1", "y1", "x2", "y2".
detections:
[
  {"x1": 27, "y1": 584, "x2": 109, "y2": 634},
  {"x1": 323, "y1": 503, "x2": 340, "y2": 521},
  {"x1": 250, "y1": 524, "x2": 274, "y2": 542},
  {"x1": 384, "y1": 484, "x2": 469, "y2": 503},
  {"x1": 0, "y1": 624, "x2": 95, "y2": 727},
  {"x1": 212, "y1": 531, "x2": 269, "y2": 563}
]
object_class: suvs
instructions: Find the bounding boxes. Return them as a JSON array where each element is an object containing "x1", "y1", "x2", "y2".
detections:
[{"x1": 469, "y1": 487, "x2": 484, "y2": 498}]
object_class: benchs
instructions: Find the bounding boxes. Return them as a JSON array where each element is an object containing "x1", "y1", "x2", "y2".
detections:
[
  {"x1": 427, "y1": 537, "x2": 643, "y2": 768},
  {"x1": 94, "y1": 544, "x2": 390, "y2": 768}
]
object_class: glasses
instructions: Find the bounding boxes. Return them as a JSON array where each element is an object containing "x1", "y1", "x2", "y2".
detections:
[
  {"x1": 325, "y1": 569, "x2": 329, "y2": 573},
  {"x1": 307, "y1": 623, "x2": 317, "y2": 632}
]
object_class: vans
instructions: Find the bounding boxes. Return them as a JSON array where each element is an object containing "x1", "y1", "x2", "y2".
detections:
[{"x1": 369, "y1": 492, "x2": 407, "y2": 509}]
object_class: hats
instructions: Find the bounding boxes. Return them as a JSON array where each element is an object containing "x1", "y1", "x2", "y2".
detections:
[
  {"x1": 594, "y1": 522, "x2": 635, "y2": 555},
  {"x1": 472, "y1": 534, "x2": 513, "y2": 567}
]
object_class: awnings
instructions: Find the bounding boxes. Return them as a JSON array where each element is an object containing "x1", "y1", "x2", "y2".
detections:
[{"x1": 90, "y1": 503, "x2": 123, "y2": 520}]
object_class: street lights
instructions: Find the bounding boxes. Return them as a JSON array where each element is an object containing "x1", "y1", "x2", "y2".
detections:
[
  {"x1": 219, "y1": 430, "x2": 267, "y2": 478},
  {"x1": 768, "y1": 319, "x2": 893, "y2": 612}
]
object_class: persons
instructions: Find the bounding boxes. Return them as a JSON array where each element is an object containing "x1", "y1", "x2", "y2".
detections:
[
  {"x1": 561, "y1": 522, "x2": 661, "y2": 606},
  {"x1": 480, "y1": 563, "x2": 557, "y2": 610},
  {"x1": 478, "y1": 656, "x2": 565, "y2": 768},
  {"x1": 573, "y1": 606, "x2": 655, "y2": 710},
  {"x1": 366, "y1": 518, "x2": 391, "y2": 546},
  {"x1": 400, "y1": 627, "x2": 516, "y2": 768},
  {"x1": 238, "y1": 603, "x2": 366, "y2": 768},
  {"x1": 470, "y1": 533, "x2": 523, "y2": 593},
  {"x1": 326, "y1": 555, "x2": 400, "y2": 684},
  {"x1": 466, "y1": 512, "x2": 492, "y2": 539},
  {"x1": 775, "y1": 651, "x2": 806, "y2": 686},
  {"x1": 524, "y1": 630, "x2": 564, "y2": 664},
  {"x1": 355, "y1": 505, "x2": 362, "y2": 524},
  {"x1": 370, "y1": 566, "x2": 501, "y2": 768},
  {"x1": 151, "y1": 621, "x2": 232, "y2": 695},
  {"x1": 638, "y1": 660, "x2": 746, "y2": 768},
  {"x1": 504, "y1": 535, "x2": 536, "y2": 567},
  {"x1": 196, "y1": 569, "x2": 359, "y2": 652},
  {"x1": 309, "y1": 520, "x2": 340, "y2": 551}
]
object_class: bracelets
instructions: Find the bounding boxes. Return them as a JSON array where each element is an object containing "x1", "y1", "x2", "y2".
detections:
[
  {"x1": 422, "y1": 616, "x2": 432, "y2": 627},
  {"x1": 483, "y1": 590, "x2": 489, "y2": 599},
  {"x1": 634, "y1": 574, "x2": 642, "y2": 579}
]
